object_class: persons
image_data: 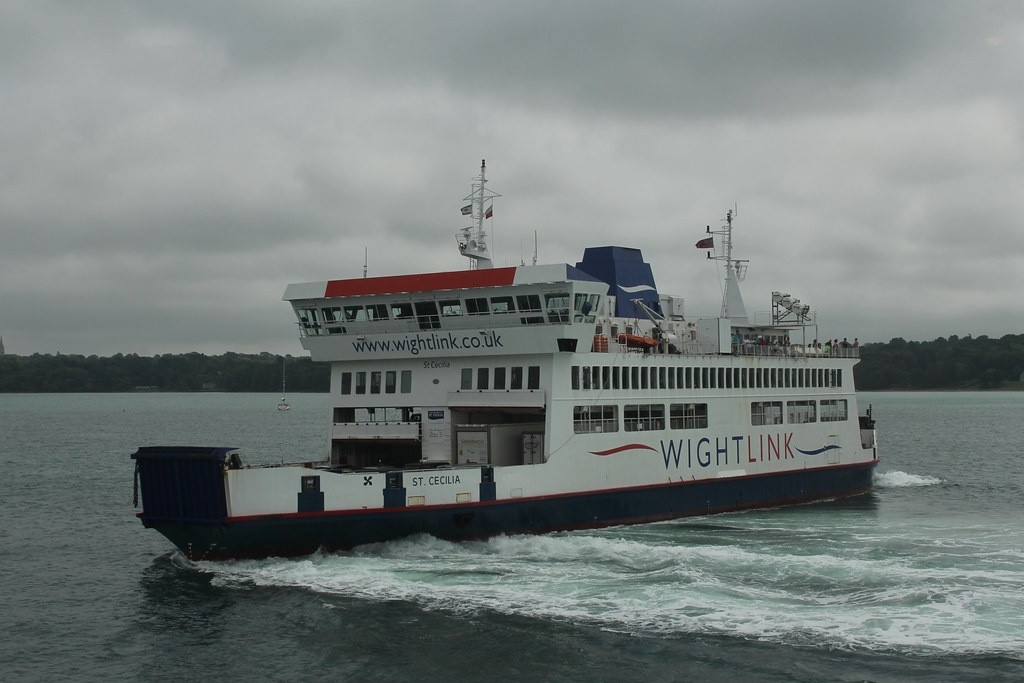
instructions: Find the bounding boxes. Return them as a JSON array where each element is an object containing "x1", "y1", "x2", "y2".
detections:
[{"x1": 732, "y1": 330, "x2": 860, "y2": 356}]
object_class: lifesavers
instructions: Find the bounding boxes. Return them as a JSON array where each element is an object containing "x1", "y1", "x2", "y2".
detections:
[{"x1": 594, "y1": 336, "x2": 609, "y2": 353}]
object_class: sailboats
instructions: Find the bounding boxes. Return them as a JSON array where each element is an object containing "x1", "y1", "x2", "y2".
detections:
[{"x1": 278, "y1": 360, "x2": 289, "y2": 412}]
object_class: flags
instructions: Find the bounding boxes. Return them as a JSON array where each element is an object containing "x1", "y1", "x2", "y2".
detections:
[
  {"x1": 461, "y1": 205, "x2": 472, "y2": 216},
  {"x1": 484, "y1": 206, "x2": 492, "y2": 219},
  {"x1": 695, "y1": 237, "x2": 713, "y2": 249}
]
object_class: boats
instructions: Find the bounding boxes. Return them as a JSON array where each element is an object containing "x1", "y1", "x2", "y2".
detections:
[
  {"x1": 616, "y1": 335, "x2": 659, "y2": 349},
  {"x1": 131, "y1": 159, "x2": 882, "y2": 563}
]
